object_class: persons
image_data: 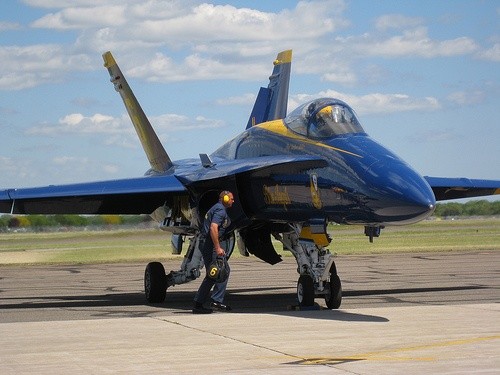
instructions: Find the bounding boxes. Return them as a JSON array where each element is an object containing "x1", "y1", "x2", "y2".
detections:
[
  {"x1": 310, "y1": 102, "x2": 335, "y2": 138},
  {"x1": 192, "y1": 190, "x2": 235, "y2": 314}
]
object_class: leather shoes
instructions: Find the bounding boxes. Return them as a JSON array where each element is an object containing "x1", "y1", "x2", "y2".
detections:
[
  {"x1": 193, "y1": 305, "x2": 212, "y2": 313},
  {"x1": 209, "y1": 303, "x2": 232, "y2": 311}
]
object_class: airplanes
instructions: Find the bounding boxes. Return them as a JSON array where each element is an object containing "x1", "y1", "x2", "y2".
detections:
[{"x1": 0, "y1": 50, "x2": 500, "y2": 308}]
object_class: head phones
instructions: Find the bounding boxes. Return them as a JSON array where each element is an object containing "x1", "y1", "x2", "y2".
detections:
[{"x1": 223, "y1": 190, "x2": 230, "y2": 203}]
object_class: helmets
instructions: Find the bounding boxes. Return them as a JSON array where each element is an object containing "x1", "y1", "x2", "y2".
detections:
[{"x1": 208, "y1": 258, "x2": 230, "y2": 283}]
